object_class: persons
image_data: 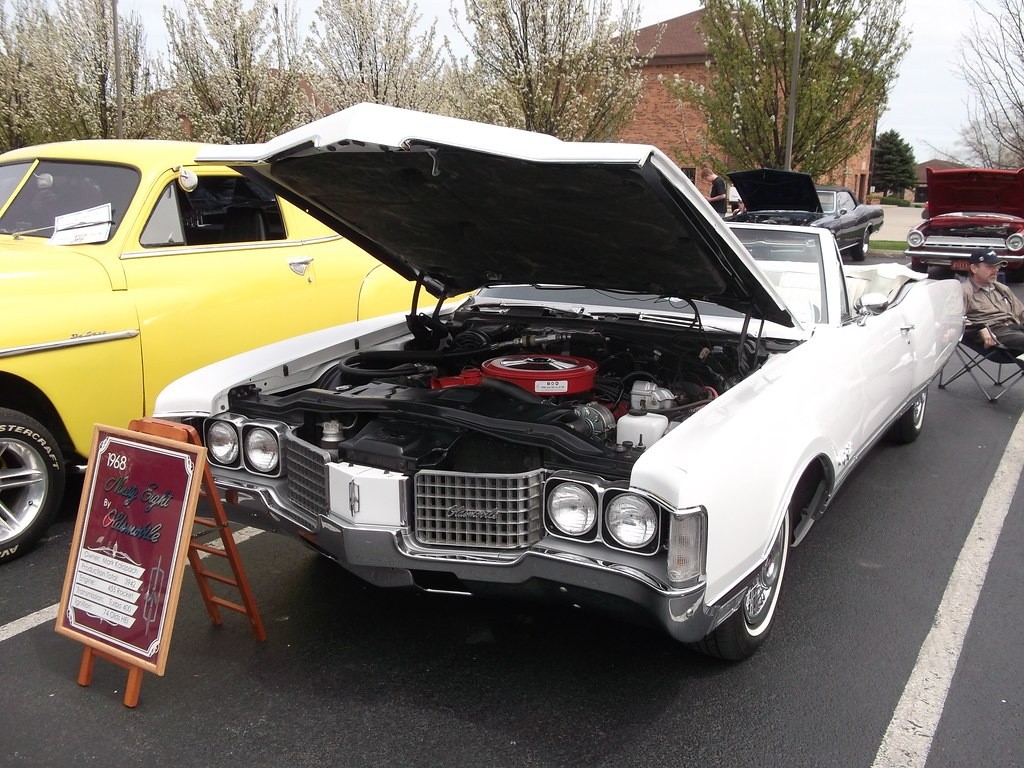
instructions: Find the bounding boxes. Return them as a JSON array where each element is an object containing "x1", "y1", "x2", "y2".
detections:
[
  {"x1": 960, "y1": 248, "x2": 1024, "y2": 362},
  {"x1": 701, "y1": 168, "x2": 727, "y2": 218},
  {"x1": 737, "y1": 197, "x2": 748, "y2": 212}
]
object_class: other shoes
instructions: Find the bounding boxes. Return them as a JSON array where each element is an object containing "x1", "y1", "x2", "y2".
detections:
[{"x1": 1015, "y1": 353, "x2": 1024, "y2": 370}]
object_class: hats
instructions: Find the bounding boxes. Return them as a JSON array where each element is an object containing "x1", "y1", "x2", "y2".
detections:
[{"x1": 970, "y1": 249, "x2": 1008, "y2": 267}]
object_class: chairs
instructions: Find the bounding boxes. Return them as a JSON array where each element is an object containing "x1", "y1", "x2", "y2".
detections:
[
  {"x1": 781, "y1": 270, "x2": 869, "y2": 320},
  {"x1": 218, "y1": 206, "x2": 273, "y2": 243},
  {"x1": 937, "y1": 272, "x2": 1024, "y2": 405}
]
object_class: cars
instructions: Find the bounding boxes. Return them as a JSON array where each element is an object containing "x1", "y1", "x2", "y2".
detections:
[
  {"x1": 903, "y1": 165, "x2": 1024, "y2": 279},
  {"x1": 716, "y1": 168, "x2": 887, "y2": 262},
  {"x1": 152, "y1": 101, "x2": 970, "y2": 663},
  {"x1": 0, "y1": 137, "x2": 479, "y2": 564}
]
